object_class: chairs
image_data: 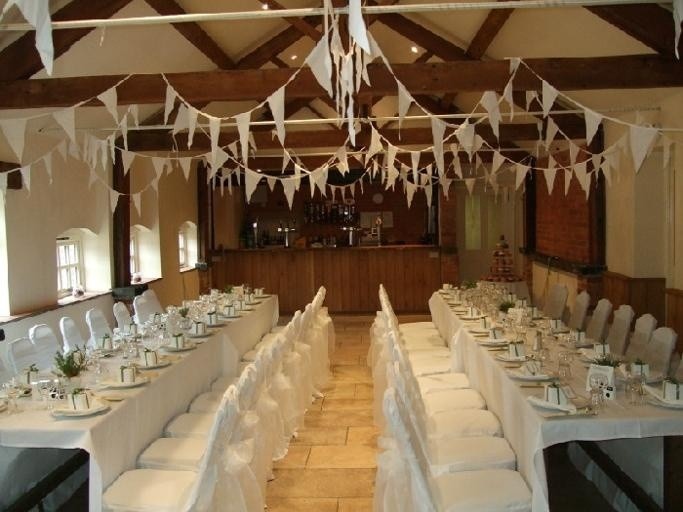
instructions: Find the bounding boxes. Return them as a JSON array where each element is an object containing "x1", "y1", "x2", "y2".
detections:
[
  {"x1": 366, "y1": 283, "x2": 534, "y2": 512},
  {"x1": 103, "y1": 287, "x2": 332, "y2": 509},
  {"x1": 539, "y1": 283, "x2": 678, "y2": 378},
  {"x1": 0, "y1": 285, "x2": 165, "y2": 371}
]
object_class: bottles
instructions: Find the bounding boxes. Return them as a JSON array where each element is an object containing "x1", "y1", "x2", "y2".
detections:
[
  {"x1": 302, "y1": 204, "x2": 357, "y2": 220},
  {"x1": 307, "y1": 232, "x2": 337, "y2": 248},
  {"x1": 237, "y1": 216, "x2": 265, "y2": 249}
]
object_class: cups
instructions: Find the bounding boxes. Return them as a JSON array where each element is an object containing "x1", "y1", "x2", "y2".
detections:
[
  {"x1": 438, "y1": 277, "x2": 682, "y2": 416},
  {"x1": 342, "y1": 228, "x2": 360, "y2": 248},
  {"x1": 276, "y1": 228, "x2": 298, "y2": 247}
]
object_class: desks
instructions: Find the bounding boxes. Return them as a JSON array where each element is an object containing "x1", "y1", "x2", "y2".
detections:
[
  {"x1": 2, "y1": 292, "x2": 279, "y2": 509},
  {"x1": 435, "y1": 285, "x2": 683, "y2": 511}
]
